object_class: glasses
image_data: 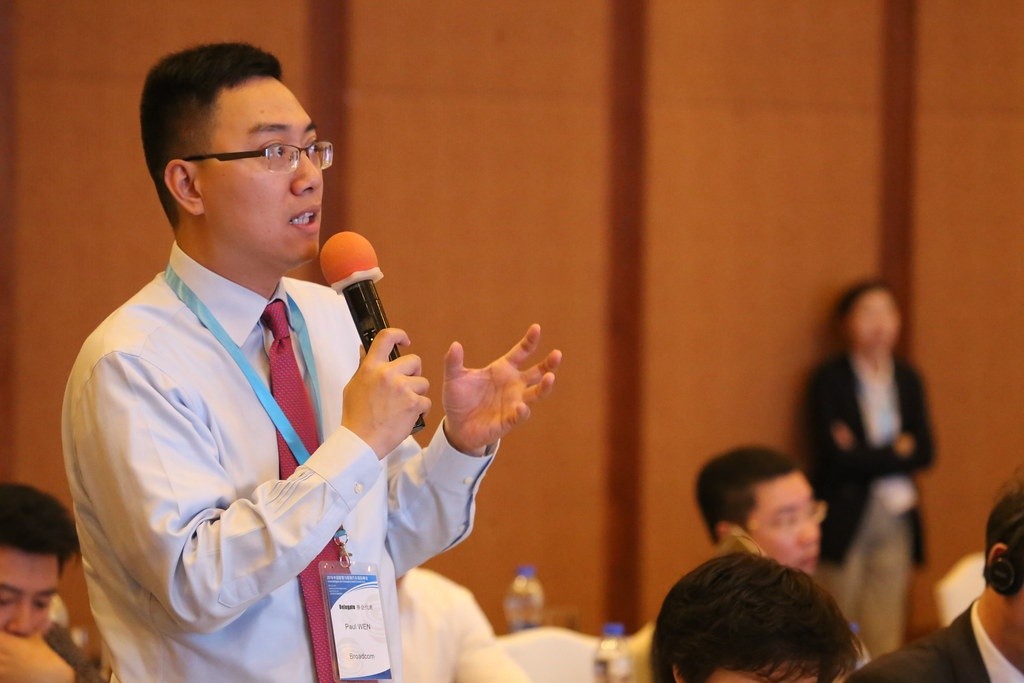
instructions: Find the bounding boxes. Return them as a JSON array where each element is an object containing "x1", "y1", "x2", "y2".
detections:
[{"x1": 181, "y1": 136, "x2": 336, "y2": 170}]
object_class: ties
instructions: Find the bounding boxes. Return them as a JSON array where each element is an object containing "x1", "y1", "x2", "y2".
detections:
[{"x1": 261, "y1": 300, "x2": 380, "y2": 683}]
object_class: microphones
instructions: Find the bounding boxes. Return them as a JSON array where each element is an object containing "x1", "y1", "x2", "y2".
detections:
[{"x1": 320, "y1": 232, "x2": 425, "y2": 434}]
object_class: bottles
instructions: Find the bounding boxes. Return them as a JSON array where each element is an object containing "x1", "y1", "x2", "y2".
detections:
[
  {"x1": 502, "y1": 566, "x2": 546, "y2": 631},
  {"x1": 592, "y1": 624, "x2": 634, "y2": 683},
  {"x1": 848, "y1": 623, "x2": 871, "y2": 670}
]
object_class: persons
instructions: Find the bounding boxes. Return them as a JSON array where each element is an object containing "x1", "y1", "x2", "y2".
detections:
[
  {"x1": 793, "y1": 279, "x2": 937, "y2": 658},
  {"x1": 61, "y1": 41, "x2": 562, "y2": 683},
  {"x1": 0, "y1": 443, "x2": 1024, "y2": 683}
]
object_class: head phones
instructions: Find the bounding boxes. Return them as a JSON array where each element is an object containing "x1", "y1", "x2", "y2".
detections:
[{"x1": 987, "y1": 526, "x2": 1024, "y2": 595}]
